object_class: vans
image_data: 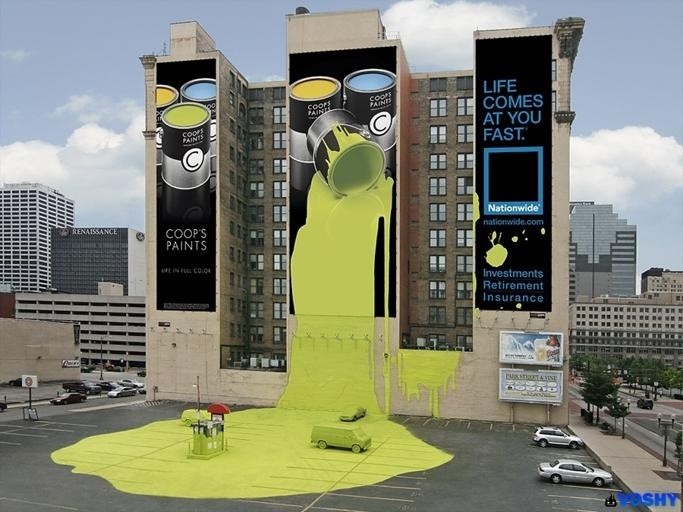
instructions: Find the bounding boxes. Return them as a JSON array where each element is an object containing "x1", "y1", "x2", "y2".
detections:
[
  {"x1": 62, "y1": 379, "x2": 101, "y2": 394},
  {"x1": 310, "y1": 422, "x2": 372, "y2": 453}
]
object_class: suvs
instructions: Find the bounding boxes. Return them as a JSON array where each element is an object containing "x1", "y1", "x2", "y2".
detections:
[
  {"x1": 532, "y1": 425, "x2": 584, "y2": 450},
  {"x1": 637, "y1": 397, "x2": 653, "y2": 410}
]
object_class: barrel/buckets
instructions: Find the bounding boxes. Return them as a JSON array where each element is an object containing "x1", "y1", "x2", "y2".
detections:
[
  {"x1": 290, "y1": 69, "x2": 397, "y2": 197},
  {"x1": 157, "y1": 77, "x2": 217, "y2": 191}
]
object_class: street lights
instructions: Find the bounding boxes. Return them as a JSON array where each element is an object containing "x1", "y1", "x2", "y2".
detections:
[
  {"x1": 654, "y1": 379, "x2": 660, "y2": 401},
  {"x1": 656, "y1": 413, "x2": 676, "y2": 466},
  {"x1": 192, "y1": 376, "x2": 200, "y2": 410},
  {"x1": 100, "y1": 335, "x2": 111, "y2": 380},
  {"x1": 617, "y1": 398, "x2": 631, "y2": 439}
]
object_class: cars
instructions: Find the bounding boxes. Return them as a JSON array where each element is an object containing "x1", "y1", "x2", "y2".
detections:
[
  {"x1": 536, "y1": 458, "x2": 611, "y2": 487},
  {"x1": 48, "y1": 392, "x2": 87, "y2": 405},
  {"x1": 94, "y1": 379, "x2": 146, "y2": 397},
  {"x1": 137, "y1": 369, "x2": 145, "y2": 377},
  {"x1": 8, "y1": 377, "x2": 21, "y2": 387},
  {"x1": 0, "y1": 399, "x2": 6, "y2": 413},
  {"x1": 572, "y1": 376, "x2": 587, "y2": 386},
  {"x1": 339, "y1": 405, "x2": 366, "y2": 422},
  {"x1": 80, "y1": 359, "x2": 126, "y2": 373}
]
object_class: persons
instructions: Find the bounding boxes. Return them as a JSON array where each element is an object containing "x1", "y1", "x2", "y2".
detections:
[
  {"x1": 546, "y1": 335, "x2": 560, "y2": 362},
  {"x1": 617, "y1": 396, "x2": 631, "y2": 410},
  {"x1": 659, "y1": 390, "x2": 663, "y2": 398}
]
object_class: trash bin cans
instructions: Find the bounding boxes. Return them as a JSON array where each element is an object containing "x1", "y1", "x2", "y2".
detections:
[{"x1": 191, "y1": 419, "x2": 222, "y2": 460}]
